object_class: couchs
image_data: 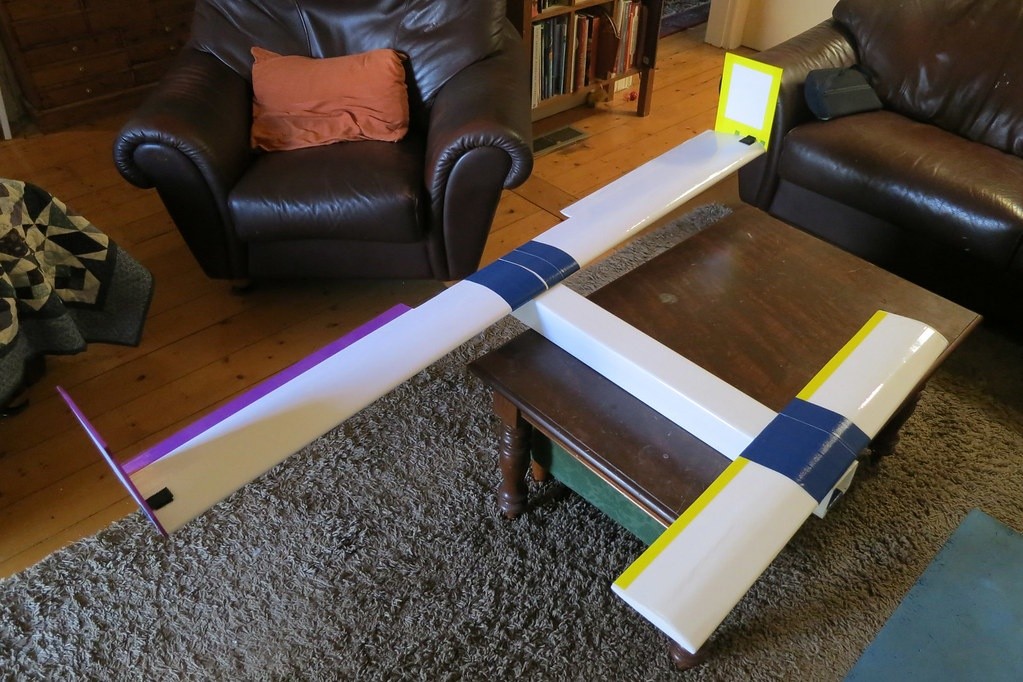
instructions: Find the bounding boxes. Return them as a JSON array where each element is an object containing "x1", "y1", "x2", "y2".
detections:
[
  {"x1": 719, "y1": 0, "x2": 1023, "y2": 348},
  {"x1": 115, "y1": 0, "x2": 535, "y2": 292}
]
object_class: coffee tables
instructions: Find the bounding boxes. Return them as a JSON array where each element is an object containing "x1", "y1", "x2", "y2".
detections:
[{"x1": 471, "y1": 207, "x2": 983, "y2": 669}]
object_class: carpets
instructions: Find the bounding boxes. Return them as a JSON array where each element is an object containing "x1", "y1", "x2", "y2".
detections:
[{"x1": 0, "y1": 204, "x2": 1023, "y2": 681}]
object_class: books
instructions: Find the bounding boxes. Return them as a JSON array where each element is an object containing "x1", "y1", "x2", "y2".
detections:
[{"x1": 531, "y1": 0, "x2": 643, "y2": 111}]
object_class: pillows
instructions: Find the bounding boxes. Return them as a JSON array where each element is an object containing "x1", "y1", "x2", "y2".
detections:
[{"x1": 250, "y1": 45, "x2": 410, "y2": 151}]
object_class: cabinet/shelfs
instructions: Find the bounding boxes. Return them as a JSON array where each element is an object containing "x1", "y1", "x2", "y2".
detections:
[
  {"x1": 506, "y1": 0, "x2": 663, "y2": 120},
  {"x1": 0, "y1": 0, "x2": 195, "y2": 134}
]
